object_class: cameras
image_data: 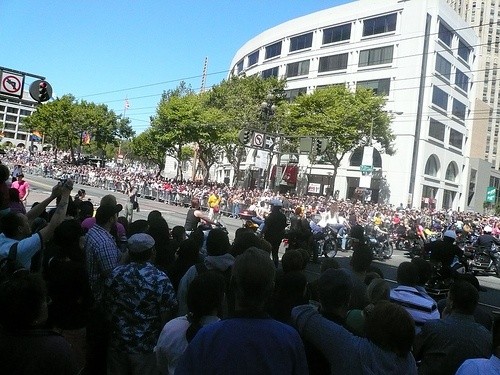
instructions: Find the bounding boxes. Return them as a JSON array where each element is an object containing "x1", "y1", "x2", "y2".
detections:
[{"x1": 61, "y1": 179, "x2": 67, "y2": 187}]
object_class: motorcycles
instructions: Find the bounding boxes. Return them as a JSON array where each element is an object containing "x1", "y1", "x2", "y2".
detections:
[
  {"x1": 366, "y1": 227, "x2": 394, "y2": 260},
  {"x1": 309, "y1": 223, "x2": 338, "y2": 259},
  {"x1": 420, "y1": 251, "x2": 474, "y2": 302},
  {"x1": 467, "y1": 246, "x2": 500, "y2": 279},
  {"x1": 185, "y1": 211, "x2": 230, "y2": 249},
  {"x1": 395, "y1": 234, "x2": 405, "y2": 249}
]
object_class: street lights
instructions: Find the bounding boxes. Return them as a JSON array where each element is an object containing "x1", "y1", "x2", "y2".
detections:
[
  {"x1": 261, "y1": 93, "x2": 277, "y2": 132},
  {"x1": 316, "y1": 139, "x2": 322, "y2": 151},
  {"x1": 362, "y1": 111, "x2": 403, "y2": 206}
]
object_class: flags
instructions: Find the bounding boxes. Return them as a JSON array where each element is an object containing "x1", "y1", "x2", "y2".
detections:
[
  {"x1": 31, "y1": 130, "x2": 42, "y2": 142},
  {"x1": 80, "y1": 129, "x2": 91, "y2": 146},
  {"x1": 0, "y1": 131, "x2": 6, "y2": 142}
]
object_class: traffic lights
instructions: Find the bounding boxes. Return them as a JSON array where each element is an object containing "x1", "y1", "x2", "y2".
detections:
[
  {"x1": 243, "y1": 130, "x2": 250, "y2": 144},
  {"x1": 38, "y1": 82, "x2": 48, "y2": 101}
]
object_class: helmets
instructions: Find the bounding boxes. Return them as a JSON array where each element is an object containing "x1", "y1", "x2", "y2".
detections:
[
  {"x1": 270, "y1": 199, "x2": 283, "y2": 206},
  {"x1": 444, "y1": 230, "x2": 457, "y2": 240},
  {"x1": 484, "y1": 225, "x2": 492, "y2": 233}
]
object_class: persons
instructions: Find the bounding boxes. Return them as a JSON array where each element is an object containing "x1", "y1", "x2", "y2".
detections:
[
  {"x1": 104, "y1": 232, "x2": 178, "y2": 375},
  {"x1": 173, "y1": 247, "x2": 310, "y2": 375},
  {"x1": 412, "y1": 279, "x2": 494, "y2": 375},
  {"x1": 0, "y1": 146, "x2": 500, "y2": 375}
]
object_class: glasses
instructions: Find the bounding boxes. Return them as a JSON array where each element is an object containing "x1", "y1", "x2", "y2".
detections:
[{"x1": 40, "y1": 296, "x2": 52, "y2": 305}]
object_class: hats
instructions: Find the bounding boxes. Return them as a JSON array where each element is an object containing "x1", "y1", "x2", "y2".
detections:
[
  {"x1": 95, "y1": 204, "x2": 122, "y2": 220},
  {"x1": 127, "y1": 232, "x2": 155, "y2": 255}
]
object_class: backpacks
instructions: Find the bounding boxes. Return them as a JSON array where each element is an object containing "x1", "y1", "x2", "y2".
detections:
[{"x1": 1, "y1": 241, "x2": 24, "y2": 283}]
